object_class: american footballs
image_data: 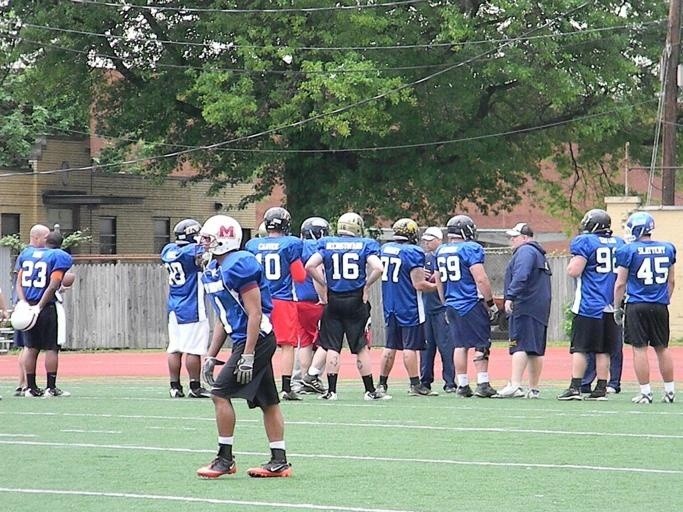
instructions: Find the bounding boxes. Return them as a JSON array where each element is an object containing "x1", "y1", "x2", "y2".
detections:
[
  {"x1": 429, "y1": 272, "x2": 435, "y2": 283},
  {"x1": 62, "y1": 272, "x2": 76, "y2": 287}
]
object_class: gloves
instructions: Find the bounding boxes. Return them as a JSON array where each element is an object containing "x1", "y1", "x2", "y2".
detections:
[
  {"x1": 613, "y1": 306, "x2": 626, "y2": 326},
  {"x1": 486, "y1": 298, "x2": 500, "y2": 325},
  {"x1": 234, "y1": 354, "x2": 255, "y2": 385},
  {"x1": 202, "y1": 357, "x2": 225, "y2": 386}
]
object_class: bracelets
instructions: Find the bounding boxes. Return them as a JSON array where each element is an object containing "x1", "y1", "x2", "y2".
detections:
[{"x1": 486, "y1": 299, "x2": 494, "y2": 308}]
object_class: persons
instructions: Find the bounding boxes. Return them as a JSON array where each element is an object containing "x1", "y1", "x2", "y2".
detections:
[
  {"x1": 159, "y1": 219, "x2": 210, "y2": 399},
  {"x1": 196, "y1": 215, "x2": 293, "y2": 479},
  {"x1": 433, "y1": 215, "x2": 497, "y2": 398},
  {"x1": 244, "y1": 207, "x2": 307, "y2": 400},
  {"x1": 491, "y1": 221, "x2": 552, "y2": 398},
  {"x1": 290, "y1": 217, "x2": 333, "y2": 395},
  {"x1": 305, "y1": 212, "x2": 382, "y2": 399},
  {"x1": 421, "y1": 227, "x2": 457, "y2": 393},
  {"x1": 556, "y1": 209, "x2": 676, "y2": 403},
  {"x1": 376, "y1": 217, "x2": 438, "y2": 397},
  {"x1": 0, "y1": 224, "x2": 72, "y2": 397}
]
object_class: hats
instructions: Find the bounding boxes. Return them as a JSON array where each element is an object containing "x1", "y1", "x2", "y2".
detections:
[
  {"x1": 45, "y1": 232, "x2": 63, "y2": 245},
  {"x1": 421, "y1": 226, "x2": 443, "y2": 241},
  {"x1": 505, "y1": 222, "x2": 533, "y2": 237}
]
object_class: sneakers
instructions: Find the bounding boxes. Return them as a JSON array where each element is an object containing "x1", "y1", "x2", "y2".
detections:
[
  {"x1": 169, "y1": 385, "x2": 185, "y2": 398},
  {"x1": 474, "y1": 382, "x2": 497, "y2": 397},
  {"x1": 188, "y1": 388, "x2": 212, "y2": 398},
  {"x1": 246, "y1": 459, "x2": 292, "y2": 477},
  {"x1": 279, "y1": 372, "x2": 338, "y2": 400},
  {"x1": 364, "y1": 384, "x2": 393, "y2": 401},
  {"x1": 525, "y1": 388, "x2": 540, "y2": 399},
  {"x1": 490, "y1": 380, "x2": 526, "y2": 398},
  {"x1": 15, "y1": 386, "x2": 71, "y2": 399},
  {"x1": 456, "y1": 384, "x2": 474, "y2": 398},
  {"x1": 557, "y1": 386, "x2": 616, "y2": 401},
  {"x1": 632, "y1": 391, "x2": 675, "y2": 403},
  {"x1": 408, "y1": 381, "x2": 456, "y2": 396},
  {"x1": 196, "y1": 456, "x2": 236, "y2": 480}
]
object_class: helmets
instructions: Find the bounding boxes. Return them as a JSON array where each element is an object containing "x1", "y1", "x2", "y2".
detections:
[
  {"x1": 301, "y1": 217, "x2": 334, "y2": 239},
  {"x1": 580, "y1": 209, "x2": 612, "y2": 238},
  {"x1": 337, "y1": 212, "x2": 365, "y2": 238},
  {"x1": 446, "y1": 214, "x2": 479, "y2": 241},
  {"x1": 200, "y1": 214, "x2": 243, "y2": 254},
  {"x1": 258, "y1": 207, "x2": 291, "y2": 237},
  {"x1": 173, "y1": 219, "x2": 202, "y2": 248},
  {"x1": 10, "y1": 308, "x2": 38, "y2": 332},
  {"x1": 392, "y1": 218, "x2": 420, "y2": 246},
  {"x1": 624, "y1": 212, "x2": 655, "y2": 242}
]
